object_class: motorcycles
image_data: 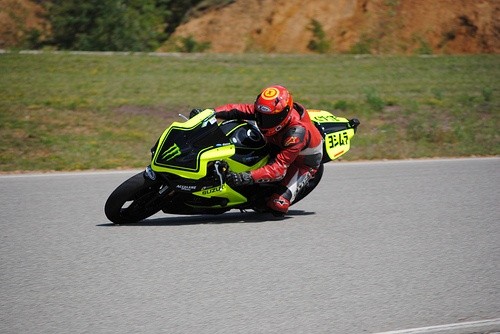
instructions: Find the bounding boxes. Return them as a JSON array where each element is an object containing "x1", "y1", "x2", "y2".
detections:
[{"x1": 104, "y1": 108, "x2": 359, "y2": 223}]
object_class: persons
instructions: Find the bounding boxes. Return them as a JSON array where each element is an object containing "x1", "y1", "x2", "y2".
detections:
[{"x1": 187, "y1": 84, "x2": 324, "y2": 217}]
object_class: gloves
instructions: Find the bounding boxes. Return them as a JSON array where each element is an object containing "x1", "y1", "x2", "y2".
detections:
[
  {"x1": 226, "y1": 169, "x2": 255, "y2": 187},
  {"x1": 189, "y1": 107, "x2": 215, "y2": 120}
]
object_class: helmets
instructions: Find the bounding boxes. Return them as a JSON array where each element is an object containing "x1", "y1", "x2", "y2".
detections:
[{"x1": 254, "y1": 86, "x2": 293, "y2": 137}]
